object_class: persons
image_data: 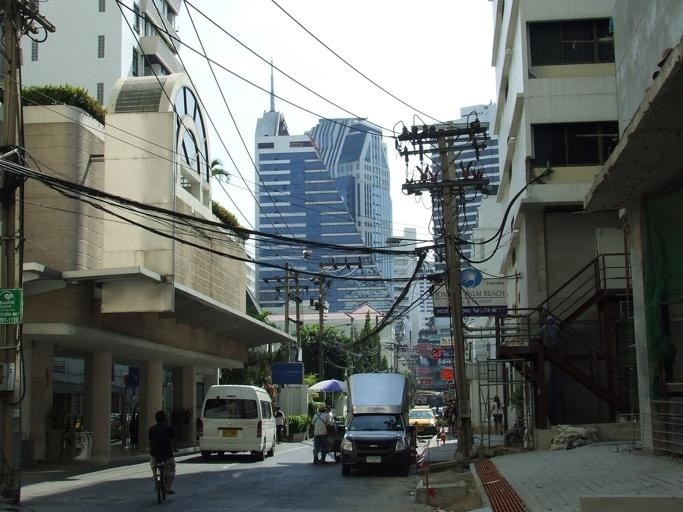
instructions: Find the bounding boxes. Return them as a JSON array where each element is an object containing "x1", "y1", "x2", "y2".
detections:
[
  {"x1": 312, "y1": 407, "x2": 329, "y2": 464},
  {"x1": 275, "y1": 407, "x2": 285, "y2": 444},
  {"x1": 491, "y1": 396, "x2": 505, "y2": 435},
  {"x1": 148, "y1": 410, "x2": 175, "y2": 493}
]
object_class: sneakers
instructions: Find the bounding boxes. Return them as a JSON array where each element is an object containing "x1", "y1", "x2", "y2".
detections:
[{"x1": 166, "y1": 490, "x2": 175, "y2": 494}]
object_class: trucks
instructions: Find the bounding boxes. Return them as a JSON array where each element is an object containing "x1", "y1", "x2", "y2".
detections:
[{"x1": 339, "y1": 371, "x2": 411, "y2": 477}]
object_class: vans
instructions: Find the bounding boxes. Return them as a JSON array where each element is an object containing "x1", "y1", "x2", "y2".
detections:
[{"x1": 197, "y1": 384, "x2": 285, "y2": 461}]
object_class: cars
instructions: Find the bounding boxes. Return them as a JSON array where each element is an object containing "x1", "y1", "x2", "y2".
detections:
[
  {"x1": 108, "y1": 411, "x2": 131, "y2": 440},
  {"x1": 431, "y1": 406, "x2": 445, "y2": 418},
  {"x1": 409, "y1": 404, "x2": 437, "y2": 437}
]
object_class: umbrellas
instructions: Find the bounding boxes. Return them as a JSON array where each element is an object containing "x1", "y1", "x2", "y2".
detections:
[{"x1": 308, "y1": 378, "x2": 347, "y2": 413}]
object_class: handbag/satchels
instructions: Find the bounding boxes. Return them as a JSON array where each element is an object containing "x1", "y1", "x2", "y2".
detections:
[{"x1": 327, "y1": 424, "x2": 335, "y2": 435}]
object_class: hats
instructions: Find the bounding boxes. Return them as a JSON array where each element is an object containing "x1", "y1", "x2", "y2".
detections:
[{"x1": 319, "y1": 403, "x2": 328, "y2": 410}]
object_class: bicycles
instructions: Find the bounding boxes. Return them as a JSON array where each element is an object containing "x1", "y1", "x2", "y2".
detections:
[
  {"x1": 146, "y1": 448, "x2": 180, "y2": 505},
  {"x1": 331, "y1": 439, "x2": 341, "y2": 462}
]
